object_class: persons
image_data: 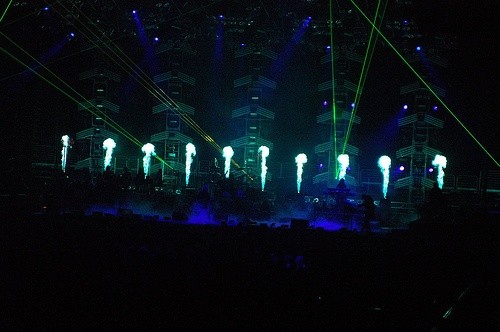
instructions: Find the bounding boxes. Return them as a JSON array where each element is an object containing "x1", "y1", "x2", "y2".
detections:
[{"x1": 85, "y1": 161, "x2": 238, "y2": 198}]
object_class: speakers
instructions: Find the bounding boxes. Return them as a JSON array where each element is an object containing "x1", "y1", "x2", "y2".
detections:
[
  {"x1": 118, "y1": 208, "x2": 133, "y2": 215},
  {"x1": 172, "y1": 212, "x2": 189, "y2": 220},
  {"x1": 290, "y1": 218, "x2": 309, "y2": 229}
]
